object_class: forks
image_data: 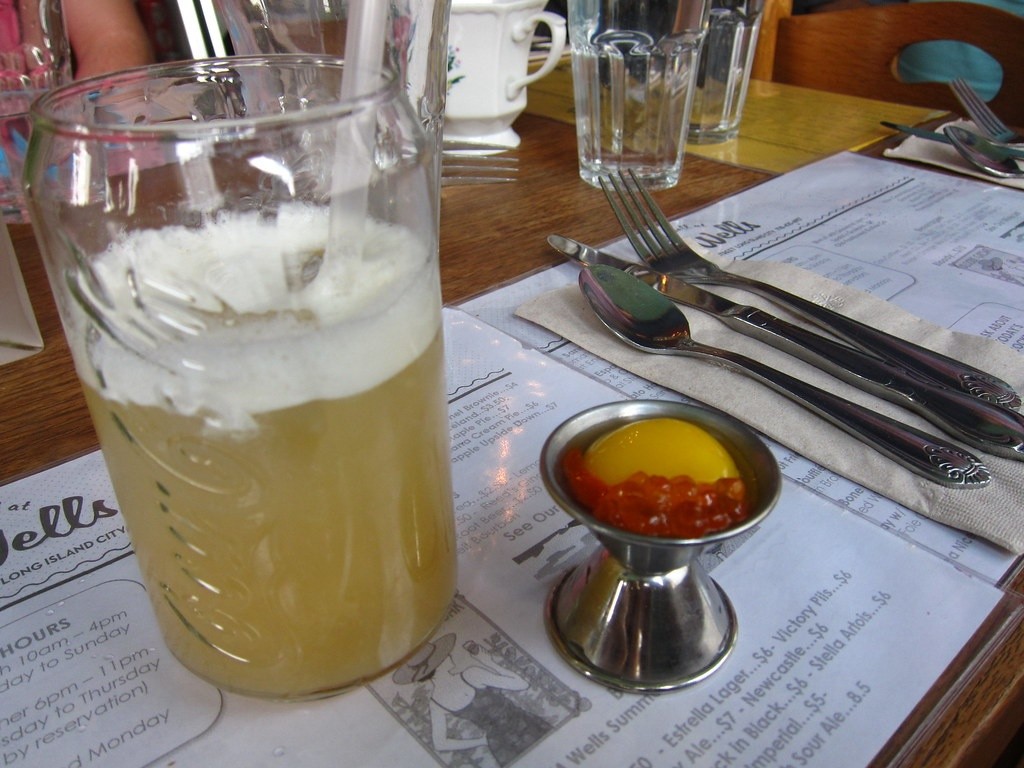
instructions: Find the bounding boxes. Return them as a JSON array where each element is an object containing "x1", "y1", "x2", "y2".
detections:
[
  {"x1": 945, "y1": 77, "x2": 1024, "y2": 143},
  {"x1": 439, "y1": 137, "x2": 521, "y2": 186},
  {"x1": 597, "y1": 168, "x2": 1024, "y2": 413}
]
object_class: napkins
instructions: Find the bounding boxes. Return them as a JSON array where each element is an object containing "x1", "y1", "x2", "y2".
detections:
[{"x1": 513, "y1": 237, "x2": 1024, "y2": 552}]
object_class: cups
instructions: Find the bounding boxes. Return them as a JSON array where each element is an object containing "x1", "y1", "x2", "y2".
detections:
[
  {"x1": 687, "y1": 0, "x2": 766, "y2": 145},
  {"x1": 566, "y1": 0, "x2": 713, "y2": 193},
  {"x1": 211, "y1": 0, "x2": 452, "y2": 201},
  {"x1": 443, "y1": 0, "x2": 567, "y2": 157},
  {"x1": 0, "y1": 0, "x2": 73, "y2": 224},
  {"x1": 539, "y1": 400, "x2": 782, "y2": 693},
  {"x1": 19, "y1": 53, "x2": 457, "y2": 702}
]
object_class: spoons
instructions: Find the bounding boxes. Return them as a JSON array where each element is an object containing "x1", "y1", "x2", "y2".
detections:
[
  {"x1": 578, "y1": 262, "x2": 992, "y2": 490},
  {"x1": 943, "y1": 125, "x2": 1024, "y2": 178}
]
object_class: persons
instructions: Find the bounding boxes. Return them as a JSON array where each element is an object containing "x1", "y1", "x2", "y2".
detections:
[{"x1": 0, "y1": 0, "x2": 158, "y2": 203}]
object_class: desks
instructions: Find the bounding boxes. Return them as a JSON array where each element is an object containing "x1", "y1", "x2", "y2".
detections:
[{"x1": 0, "y1": 57, "x2": 1024, "y2": 768}]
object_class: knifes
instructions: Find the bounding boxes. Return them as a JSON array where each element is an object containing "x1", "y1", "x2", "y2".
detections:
[
  {"x1": 879, "y1": 120, "x2": 1024, "y2": 161},
  {"x1": 546, "y1": 233, "x2": 1024, "y2": 463}
]
